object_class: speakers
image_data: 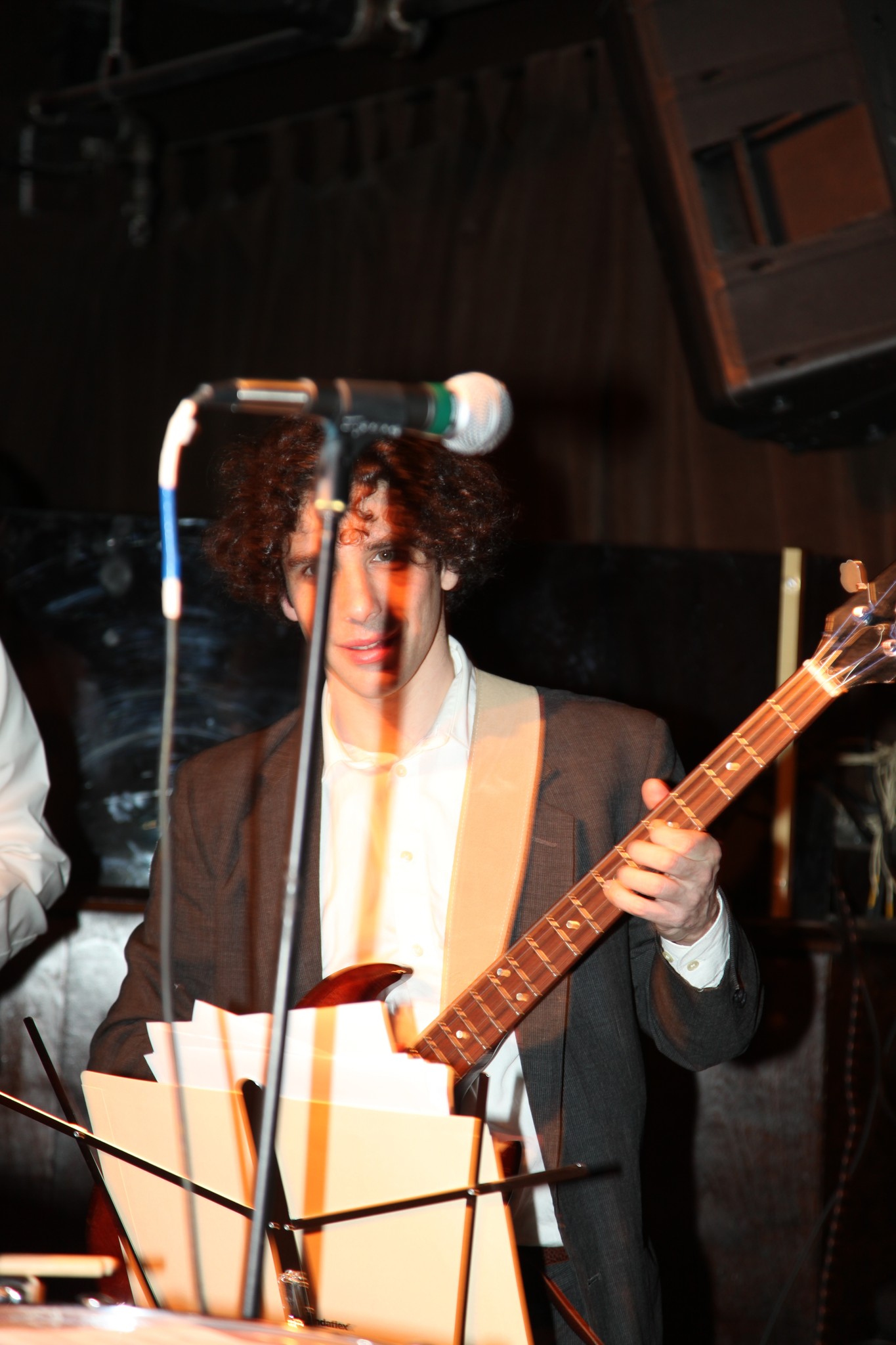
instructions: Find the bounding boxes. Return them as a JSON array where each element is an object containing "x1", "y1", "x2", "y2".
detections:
[{"x1": 599, "y1": 0, "x2": 896, "y2": 448}]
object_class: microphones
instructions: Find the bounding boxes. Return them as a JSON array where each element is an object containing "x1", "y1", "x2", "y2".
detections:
[{"x1": 205, "y1": 364, "x2": 514, "y2": 461}]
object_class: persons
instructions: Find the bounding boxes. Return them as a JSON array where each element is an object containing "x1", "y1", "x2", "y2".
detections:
[
  {"x1": 0, "y1": 644, "x2": 72, "y2": 972},
  {"x1": 88, "y1": 416, "x2": 764, "y2": 1345}
]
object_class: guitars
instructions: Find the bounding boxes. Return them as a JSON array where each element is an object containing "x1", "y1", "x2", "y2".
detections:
[{"x1": 90, "y1": 557, "x2": 896, "y2": 1300}]
